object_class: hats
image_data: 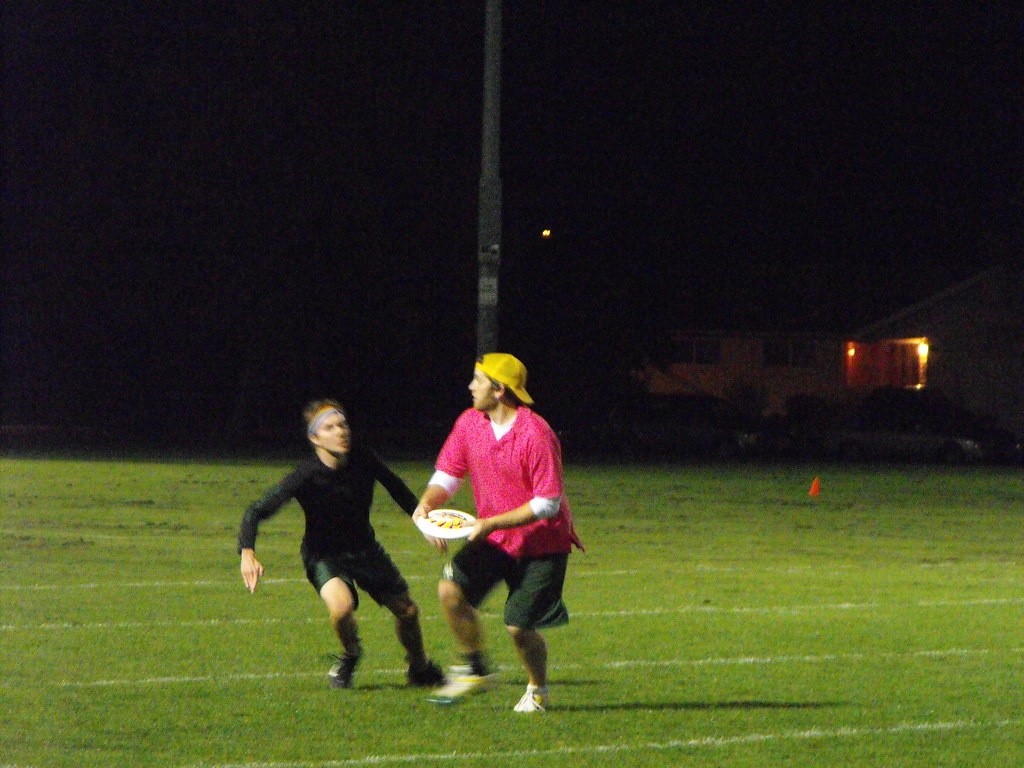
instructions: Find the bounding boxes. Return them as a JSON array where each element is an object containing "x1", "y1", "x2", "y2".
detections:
[{"x1": 477, "y1": 352, "x2": 535, "y2": 405}]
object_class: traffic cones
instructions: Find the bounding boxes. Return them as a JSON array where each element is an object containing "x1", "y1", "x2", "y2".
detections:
[{"x1": 808, "y1": 477, "x2": 821, "y2": 497}]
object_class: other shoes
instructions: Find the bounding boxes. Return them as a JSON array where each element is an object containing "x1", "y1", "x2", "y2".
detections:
[
  {"x1": 325, "y1": 651, "x2": 362, "y2": 688},
  {"x1": 403, "y1": 657, "x2": 446, "y2": 686}
]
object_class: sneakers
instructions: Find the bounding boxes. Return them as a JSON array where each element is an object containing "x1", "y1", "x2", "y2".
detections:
[
  {"x1": 423, "y1": 666, "x2": 496, "y2": 707},
  {"x1": 513, "y1": 684, "x2": 550, "y2": 713}
]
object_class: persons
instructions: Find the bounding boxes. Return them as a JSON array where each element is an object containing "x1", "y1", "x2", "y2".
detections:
[
  {"x1": 238, "y1": 397, "x2": 450, "y2": 688},
  {"x1": 410, "y1": 353, "x2": 586, "y2": 712}
]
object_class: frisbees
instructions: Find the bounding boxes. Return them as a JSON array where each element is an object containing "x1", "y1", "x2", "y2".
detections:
[{"x1": 418, "y1": 509, "x2": 478, "y2": 541}]
